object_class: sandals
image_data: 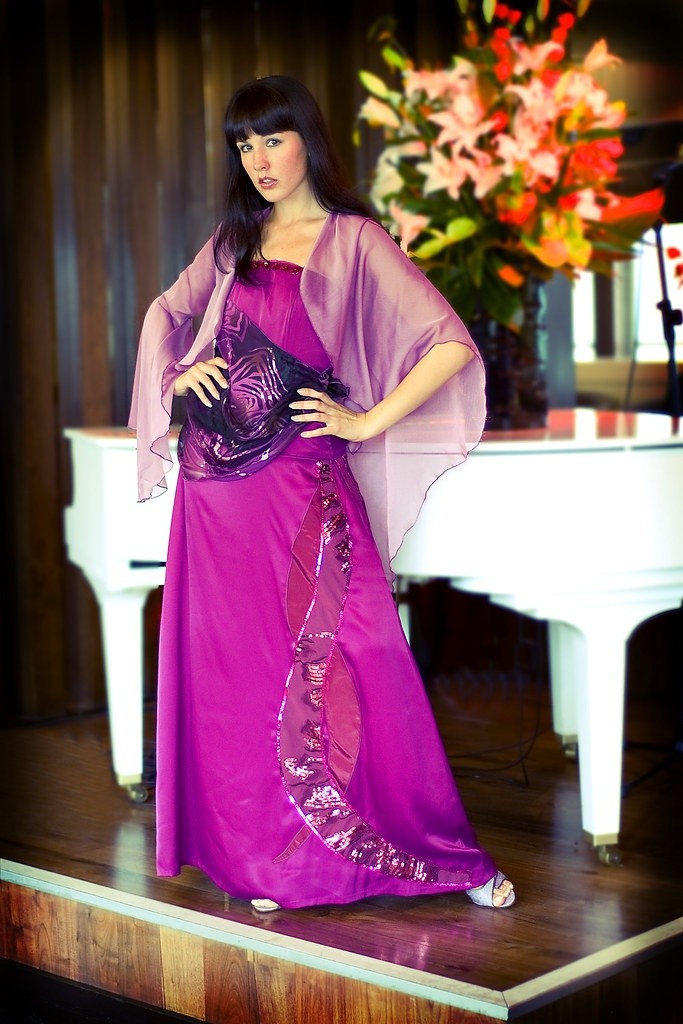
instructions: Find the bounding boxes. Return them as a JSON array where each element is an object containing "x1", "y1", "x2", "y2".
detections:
[{"x1": 467, "y1": 870, "x2": 516, "y2": 907}]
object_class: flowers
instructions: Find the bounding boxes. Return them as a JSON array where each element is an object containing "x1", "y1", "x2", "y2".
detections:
[{"x1": 353, "y1": 0, "x2": 683, "y2": 289}]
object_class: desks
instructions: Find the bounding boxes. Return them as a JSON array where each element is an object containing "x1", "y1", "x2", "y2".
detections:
[{"x1": 63, "y1": 409, "x2": 683, "y2": 863}]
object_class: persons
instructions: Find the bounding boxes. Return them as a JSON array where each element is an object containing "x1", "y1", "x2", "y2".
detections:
[{"x1": 126, "y1": 73, "x2": 519, "y2": 911}]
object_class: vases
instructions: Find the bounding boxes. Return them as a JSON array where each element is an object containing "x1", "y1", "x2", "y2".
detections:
[
  {"x1": 459, "y1": 315, "x2": 503, "y2": 433},
  {"x1": 493, "y1": 261, "x2": 547, "y2": 431}
]
